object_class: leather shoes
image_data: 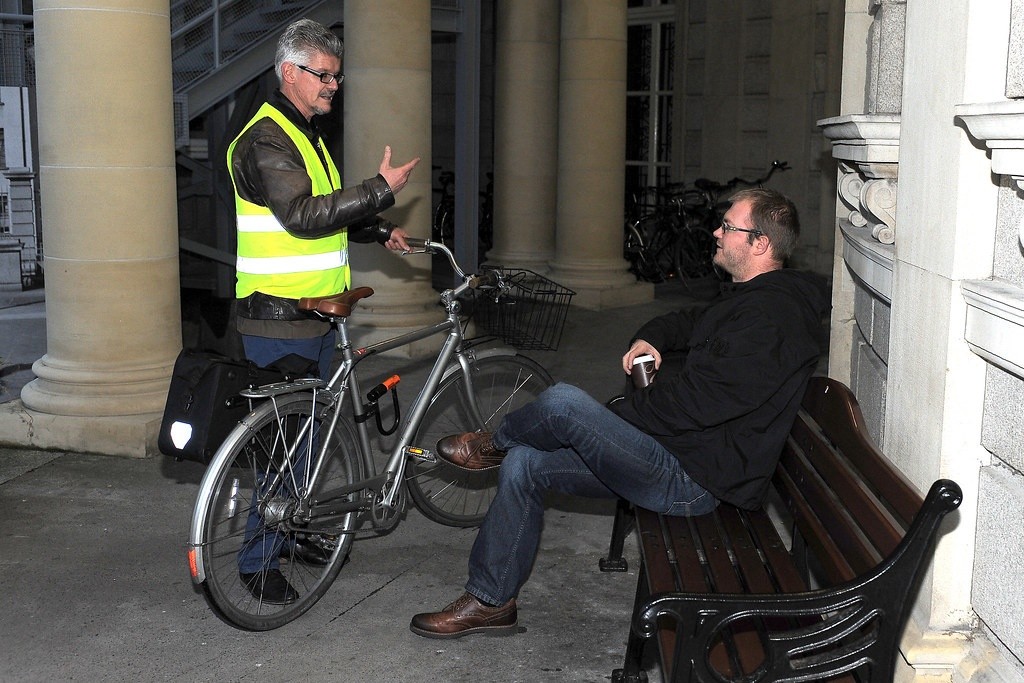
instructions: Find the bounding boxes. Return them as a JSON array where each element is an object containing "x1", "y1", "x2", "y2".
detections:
[
  {"x1": 434, "y1": 432, "x2": 508, "y2": 471},
  {"x1": 239, "y1": 569, "x2": 299, "y2": 605},
  {"x1": 278, "y1": 540, "x2": 351, "y2": 567},
  {"x1": 410, "y1": 591, "x2": 518, "y2": 639}
]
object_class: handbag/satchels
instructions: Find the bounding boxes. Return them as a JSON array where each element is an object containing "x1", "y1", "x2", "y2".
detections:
[{"x1": 158, "y1": 346, "x2": 320, "y2": 474}]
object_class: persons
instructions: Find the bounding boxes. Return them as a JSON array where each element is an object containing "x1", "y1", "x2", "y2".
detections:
[
  {"x1": 409, "y1": 183, "x2": 825, "y2": 641},
  {"x1": 227, "y1": 17, "x2": 423, "y2": 605}
]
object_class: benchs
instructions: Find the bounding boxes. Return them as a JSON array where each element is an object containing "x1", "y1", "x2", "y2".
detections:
[{"x1": 597, "y1": 376, "x2": 963, "y2": 683}]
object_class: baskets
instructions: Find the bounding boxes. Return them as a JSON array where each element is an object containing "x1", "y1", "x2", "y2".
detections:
[{"x1": 473, "y1": 266, "x2": 578, "y2": 353}]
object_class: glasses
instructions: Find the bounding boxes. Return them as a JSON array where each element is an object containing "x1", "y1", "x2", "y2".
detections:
[
  {"x1": 297, "y1": 65, "x2": 345, "y2": 85},
  {"x1": 722, "y1": 221, "x2": 762, "y2": 236}
]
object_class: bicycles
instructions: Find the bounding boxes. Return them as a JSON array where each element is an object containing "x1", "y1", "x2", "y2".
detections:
[
  {"x1": 431, "y1": 166, "x2": 494, "y2": 260},
  {"x1": 626, "y1": 159, "x2": 793, "y2": 305},
  {"x1": 184, "y1": 237, "x2": 578, "y2": 632}
]
